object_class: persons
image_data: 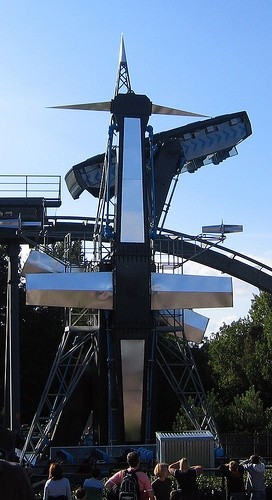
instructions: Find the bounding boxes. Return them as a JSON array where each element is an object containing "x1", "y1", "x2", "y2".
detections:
[
  {"x1": 104, "y1": 453, "x2": 155, "y2": 500},
  {"x1": 43, "y1": 463, "x2": 72, "y2": 500},
  {"x1": 151, "y1": 463, "x2": 172, "y2": 500},
  {"x1": 239, "y1": 455, "x2": 266, "y2": 500},
  {"x1": 0, "y1": 425, "x2": 35, "y2": 500},
  {"x1": 168, "y1": 458, "x2": 202, "y2": 500},
  {"x1": 219, "y1": 459, "x2": 244, "y2": 500}
]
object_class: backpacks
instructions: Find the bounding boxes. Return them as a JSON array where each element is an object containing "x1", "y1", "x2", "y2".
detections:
[{"x1": 118, "y1": 469, "x2": 141, "y2": 500}]
